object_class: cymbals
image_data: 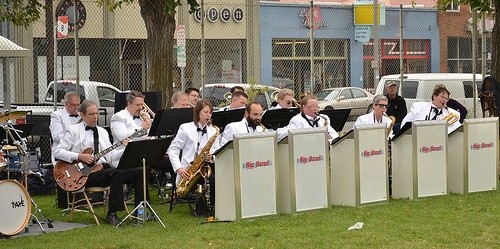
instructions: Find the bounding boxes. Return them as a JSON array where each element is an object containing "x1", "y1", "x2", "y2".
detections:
[{"x1": 0, "y1": 110, "x2": 28, "y2": 123}]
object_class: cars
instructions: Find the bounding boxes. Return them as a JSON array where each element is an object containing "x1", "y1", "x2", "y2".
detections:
[
  {"x1": 197, "y1": 84, "x2": 300, "y2": 108},
  {"x1": 313, "y1": 87, "x2": 382, "y2": 115}
]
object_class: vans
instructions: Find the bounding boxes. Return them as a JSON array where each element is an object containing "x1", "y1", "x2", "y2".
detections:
[{"x1": 377, "y1": 73, "x2": 494, "y2": 117}]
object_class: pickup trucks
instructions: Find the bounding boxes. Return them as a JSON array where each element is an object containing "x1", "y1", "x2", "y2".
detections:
[{"x1": 2, "y1": 79, "x2": 157, "y2": 127}]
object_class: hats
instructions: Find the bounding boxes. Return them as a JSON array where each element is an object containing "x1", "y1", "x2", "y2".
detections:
[
  {"x1": 387, "y1": 80, "x2": 398, "y2": 88},
  {"x1": 432, "y1": 84, "x2": 445, "y2": 99}
]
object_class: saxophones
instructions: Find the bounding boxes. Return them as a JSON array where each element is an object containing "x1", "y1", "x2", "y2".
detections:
[
  {"x1": 382, "y1": 111, "x2": 395, "y2": 138},
  {"x1": 439, "y1": 103, "x2": 459, "y2": 126},
  {"x1": 257, "y1": 119, "x2": 266, "y2": 132},
  {"x1": 173, "y1": 119, "x2": 220, "y2": 198},
  {"x1": 315, "y1": 112, "x2": 328, "y2": 129}
]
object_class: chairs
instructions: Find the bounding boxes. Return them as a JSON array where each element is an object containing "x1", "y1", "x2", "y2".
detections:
[
  {"x1": 164, "y1": 156, "x2": 214, "y2": 218},
  {"x1": 69, "y1": 183, "x2": 133, "y2": 226}
]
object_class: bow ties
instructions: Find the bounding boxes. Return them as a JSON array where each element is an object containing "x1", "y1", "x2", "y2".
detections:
[
  {"x1": 197, "y1": 127, "x2": 207, "y2": 134},
  {"x1": 133, "y1": 114, "x2": 140, "y2": 120},
  {"x1": 68, "y1": 114, "x2": 79, "y2": 118},
  {"x1": 85, "y1": 125, "x2": 97, "y2": 132},
  {"x1": 313, "y1": 117, "x2": 320, "y2": 126},
  {"x1": 248, "y1": 123, "x2": 256, "y2": 129}
]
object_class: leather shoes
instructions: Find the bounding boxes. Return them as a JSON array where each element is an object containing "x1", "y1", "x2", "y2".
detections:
[
  {"x1": 134, "y1": 212, "x2": 157, "y2": 223},
  {"x1": 104, "y1": 212, "x2": 124, "y2": 227}
]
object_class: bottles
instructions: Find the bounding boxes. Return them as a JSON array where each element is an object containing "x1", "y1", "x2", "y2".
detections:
[{"x1": 138, "y1": 202, "x2": 144, "y2": 225}]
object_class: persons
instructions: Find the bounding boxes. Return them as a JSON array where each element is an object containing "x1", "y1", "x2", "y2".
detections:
[
  {"x1": 353, "y1": 81, "x2": 407, "y2": 132},
  {"x1": 167, "y1": 100, "x2": 221, "y2": 216},
  {"x1": 49, "y1": 92, "x2": 82, "y2": 208},
  {"x1": 54, "y1": 100, "x2": 150, "y2": 224},
  {"x1": 170, "y1": 88, "x2": 199, "y2": 108},
  {"x1": 110, "y1": 90, "x2": 168, "y2": 188},
  {"x1": 400, "y1": 84, "x2": 467, "y2": 128},
  {"x1": 269, "y1": 88, "x2": 338, "y2": 142},
  {"x1": 208, "y1": 85, "x2": 269, "y2": 153}
]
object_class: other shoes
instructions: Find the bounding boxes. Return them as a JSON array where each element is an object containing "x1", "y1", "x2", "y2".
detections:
[{"x1": 196, "y1": 204, "x2": 215, "y2": 217}]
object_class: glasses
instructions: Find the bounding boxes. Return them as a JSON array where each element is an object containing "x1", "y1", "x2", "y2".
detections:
[
  {"x1": 437, "y1": 94, "x2": 449, "y2": 101},
  {"x1": 304, "y1": 104, "x2": 318, "y2": 110},
  {"x1": 281, "y1": 99, "x2": 295, "y2": 105},
  {"x1": 374, "y1": 103, "x2": 389, "y2": 108}
]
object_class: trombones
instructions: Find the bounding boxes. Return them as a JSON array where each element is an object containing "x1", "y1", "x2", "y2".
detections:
[
  {"x1": 75, "y1": 109, "x2": 80, "y2": 117},
  {"x1": 223, "y1": 92, "x2": 233, "y2": 103},
  {"x1": 139, "y1": 102, "x2": 156, "y2": 126},
  {"x1": 270, "y1": 90, "x2": 301, "y2": 108}
]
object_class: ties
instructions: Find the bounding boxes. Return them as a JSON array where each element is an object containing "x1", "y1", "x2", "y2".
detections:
[{"x1": 431, "y1": 106, "x2": 443, "y2": 120}]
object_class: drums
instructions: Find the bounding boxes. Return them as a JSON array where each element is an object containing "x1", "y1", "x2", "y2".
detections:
[
  {"x1": 0, "y1": 177, "x2": 31, "y2": 236},
  {"x1": 0, "y1": 150, "x2": 9, "y2": 172}
]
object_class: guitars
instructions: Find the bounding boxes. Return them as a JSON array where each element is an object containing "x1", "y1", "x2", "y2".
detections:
[{"x1": 54, "y1": 128, "x2": 147, "y2": 192}]
